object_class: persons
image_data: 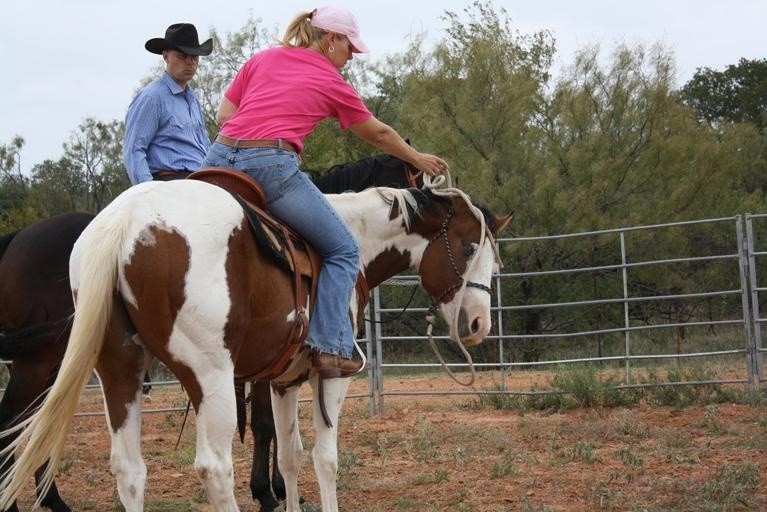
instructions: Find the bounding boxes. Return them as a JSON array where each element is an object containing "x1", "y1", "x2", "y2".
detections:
[
  {"x1": 118, "y1": 19, "x2": 221, "y2": 185},
  {"x1": 194, "y1": 2, "x2": 451, "y2": 381}
]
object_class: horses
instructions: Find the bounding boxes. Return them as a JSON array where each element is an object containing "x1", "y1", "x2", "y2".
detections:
[
  {"x1": 0, "y1": 138, "x2": 447, "y2": 512},
  {"x1": 0, "y1": 174, "x2": 515, "y2": 512}
]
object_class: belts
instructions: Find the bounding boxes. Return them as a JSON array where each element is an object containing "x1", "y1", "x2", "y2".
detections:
[
  {"x1": 151, "y1": 166, "x2": 193, "y2": 181},
  {"x1": 213, "y1": 131, "x2": 299, "y2": 152}
]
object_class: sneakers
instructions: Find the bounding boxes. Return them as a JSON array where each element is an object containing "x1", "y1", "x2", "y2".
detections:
[{"x1": 314, "y1": 346, "x2": 373, "y2": 380}]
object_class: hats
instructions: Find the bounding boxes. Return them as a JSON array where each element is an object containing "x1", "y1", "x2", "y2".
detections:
[
  {"x1": 309, "y1": 5, "x2": 370, "y2": 55},
  {"x1": 145, "y1": 22, "x2": 215, "y2": 57}
]
